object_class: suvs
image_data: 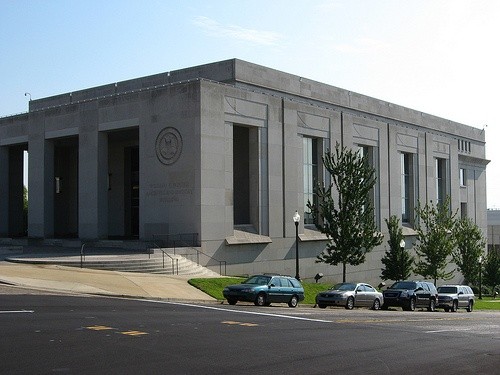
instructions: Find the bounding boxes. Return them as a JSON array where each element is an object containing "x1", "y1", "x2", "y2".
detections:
[{"x1": 223, "y1": 272, "x2": 305, "y2": 307}]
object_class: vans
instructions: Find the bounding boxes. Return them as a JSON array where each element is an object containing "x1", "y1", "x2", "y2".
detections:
[
  {"x1": 383, "y1": 281, "x2": 439, "y2": 311},
  {"x1": 436, "y1": 284, "x2": 475, "y2": 312}
]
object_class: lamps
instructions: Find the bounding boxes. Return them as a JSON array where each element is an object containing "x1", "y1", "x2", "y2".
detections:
[
  {"x1": 314, "y1": 273, "x2": 324, "y2": 283},
  {"x1": 377, "y1": 282, "x2": 386, "y2": 290},
  {"x1": 493, "y1": 292, "x2": 498, "y2": 298}
]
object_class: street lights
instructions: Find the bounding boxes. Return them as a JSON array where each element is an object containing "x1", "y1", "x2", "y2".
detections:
[
  {"x1": 293, "y1": 210, "x2": 301, "y2": 281},
  {"x1": 477, "y1": 254, "x2": 482, "y2": 299},
  {"x1": 400, "y1": 238, "x2": 405, "y2": 281}
]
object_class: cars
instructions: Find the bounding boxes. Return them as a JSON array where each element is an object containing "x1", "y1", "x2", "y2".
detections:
[{"x1": 316, "y1": 282, "x2": 384, "y2": 310}]
